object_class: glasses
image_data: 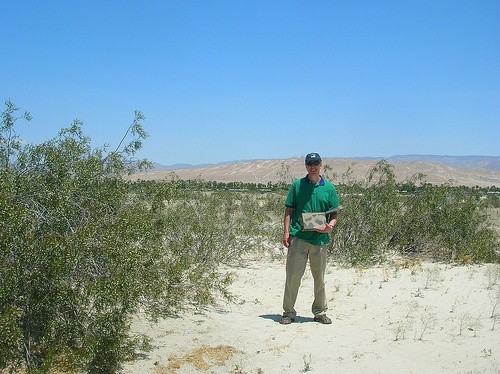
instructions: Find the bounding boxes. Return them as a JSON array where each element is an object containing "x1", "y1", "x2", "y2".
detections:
[{"x1": 305, "y1": 161, "x2": 321, "y2": 166}]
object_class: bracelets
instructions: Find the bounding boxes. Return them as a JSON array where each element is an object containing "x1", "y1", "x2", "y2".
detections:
[{"x1": 329, "y1": 225, "x2": 334, "y2": 228}]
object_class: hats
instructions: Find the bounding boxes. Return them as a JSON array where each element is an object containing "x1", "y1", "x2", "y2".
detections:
[{"x1": 305, "y1": 153, "x2": 322, "y2": 163}]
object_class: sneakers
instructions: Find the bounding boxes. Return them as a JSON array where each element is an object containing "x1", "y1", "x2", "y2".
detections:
[
  {"x1": 281, "y1": 316, "x2": 294, "y2": 325},
  {"x1": 314, "y1": 315, "x2": 332, "y2": 324}
]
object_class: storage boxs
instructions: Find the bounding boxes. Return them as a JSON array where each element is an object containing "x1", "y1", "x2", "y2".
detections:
[{"x1": 302, "y1": 203, "x2": 348, "y2": 230}]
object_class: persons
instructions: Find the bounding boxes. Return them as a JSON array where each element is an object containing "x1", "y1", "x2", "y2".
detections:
[{"x1": 279, "y1": 153, "x2": 340, "y2": 325}]
